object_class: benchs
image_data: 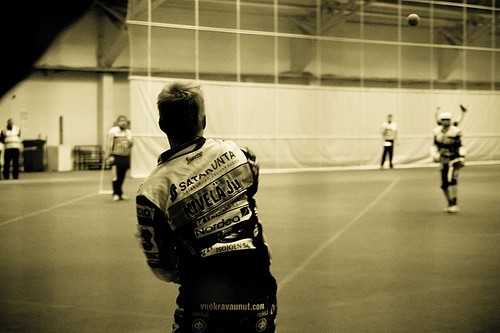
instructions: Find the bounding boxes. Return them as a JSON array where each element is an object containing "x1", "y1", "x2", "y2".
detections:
[{"x1": 74, "y1": 145, "x2": 104, "y2": 171}]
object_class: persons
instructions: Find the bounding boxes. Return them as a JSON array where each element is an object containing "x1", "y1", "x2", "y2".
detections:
[
  {"x1": 0, "y1": 118, "x2": 21, "y2": 180},
  {"x1": 380, "y1": 113, "x2": 399, "y2": 171},
  {"x1": 433, "y1": 112, "x2": 466, "y2": 213},
  {"x1": 435, "y1": 106, "x2": 467, "y2": 126},
  {"x1": 105, "y1": 115, "x2": 134, "y2": 201},
  {"x1": 137, "y1": 81, "x2": 278, "y2": 333}
]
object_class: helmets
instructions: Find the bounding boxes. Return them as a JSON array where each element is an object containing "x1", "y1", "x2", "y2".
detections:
[{"x1": 440, "y1": 111, "x2": 452, "y2": 121}]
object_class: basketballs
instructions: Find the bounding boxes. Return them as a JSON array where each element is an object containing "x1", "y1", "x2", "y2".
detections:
[{"x1": 407, "y1": 13, "x2": 420, "y2": 26}]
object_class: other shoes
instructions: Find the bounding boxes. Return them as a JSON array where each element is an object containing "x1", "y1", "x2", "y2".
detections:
[
  {"x1": 442, "y1": 206, "x2": 450, "y2": 212},
  {"x1": 112, "y1": 195, "x2": 119, "y2": 201},
  {"x1": 121, "y1": 195, "x2": 129, "y2": 200},
  {"x1": 450, "y1": 205, "x2": 460, "y2": 213}
]
